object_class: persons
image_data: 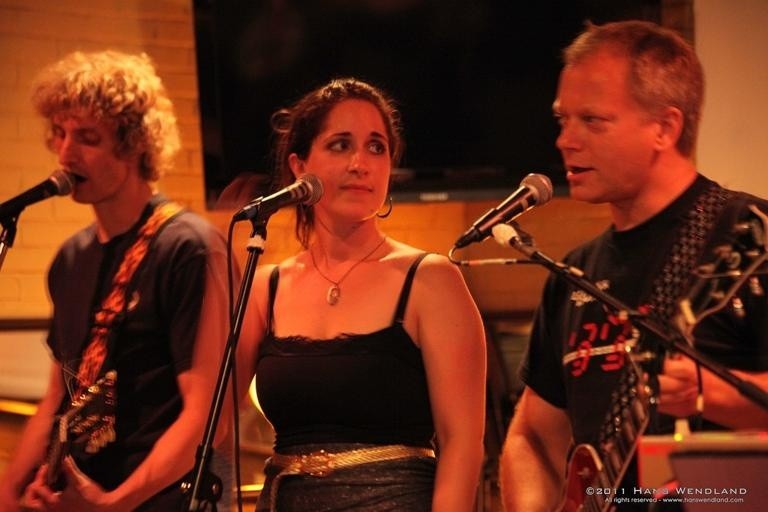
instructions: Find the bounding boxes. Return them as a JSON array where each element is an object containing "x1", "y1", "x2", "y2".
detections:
[
  {"x1": 496, "y1": 18, "x2": 767, "y2": 511},
  {"x1": 209, "y1": 77, "x2": 488, "y2": 512},
  {"x1": 0, "y1": 52, "x2": 243, "y2": 512}
]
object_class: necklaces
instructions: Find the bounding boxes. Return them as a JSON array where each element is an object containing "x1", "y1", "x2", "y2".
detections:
[{"x1": 310, "y1": 235, "x2": 387, "y2": 305}]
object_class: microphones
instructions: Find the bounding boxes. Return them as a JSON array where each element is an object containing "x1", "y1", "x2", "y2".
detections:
[
  {"x1": 0, "y1": 167, "x2": 78, "y2": 225},
  {"x1": 234, "y1": 173, "x2": 324, "y2": 227},
  {"x1": 453, "y1": 171, "x2": 554, "y2": 248}
]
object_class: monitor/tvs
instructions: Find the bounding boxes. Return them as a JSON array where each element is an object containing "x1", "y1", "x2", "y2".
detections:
[{"x1": 192, "y1": 0, "x2": 665, "y2": 212}]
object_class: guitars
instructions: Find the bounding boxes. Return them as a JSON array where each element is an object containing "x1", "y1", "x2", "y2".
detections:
[{"x1": 554, "y1": 206, "x2": 768, "y2": 512}]
